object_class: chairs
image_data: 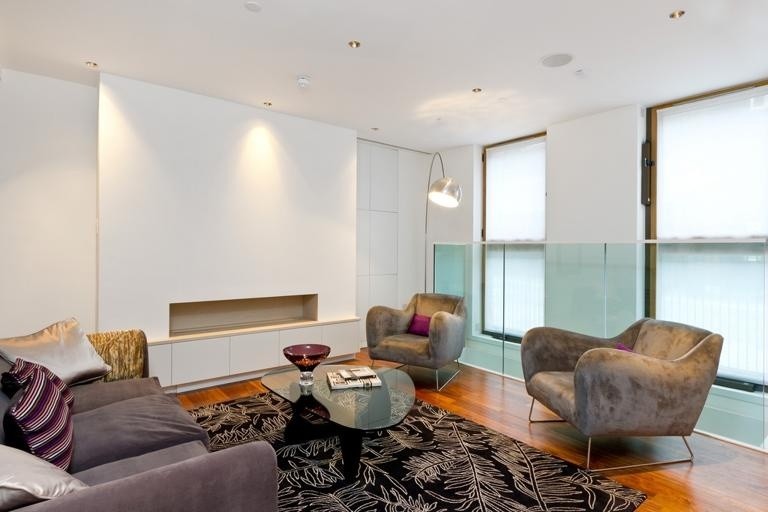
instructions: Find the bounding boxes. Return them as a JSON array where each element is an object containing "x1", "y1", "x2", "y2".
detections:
[
  {"x1": 365, "y1": 292, "x2": 469, "y2": 392},
  {"x1": 520, "y1": 318, "x2": 724, "y2": 472}
]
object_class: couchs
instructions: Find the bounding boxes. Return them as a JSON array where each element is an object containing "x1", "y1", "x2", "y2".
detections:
[{"x1": 0, "y1": 330, "x2": 281, "y2": 511}]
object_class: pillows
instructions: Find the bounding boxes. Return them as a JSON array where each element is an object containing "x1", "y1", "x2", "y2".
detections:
[
  {"x1": 615, "y1": 342, "x2": 633, "y2": 353},
  {"x1": 0, "y1": 317, "x2": 114, "y2": 388},
  {"x1": 0, "y1": 442, "x2": 90, "y2": 511},
  {"x1": 406, "y1": 313, "x2": 431, "y2": 337},
  {"x1": 2, "y1": 357, "x2": 76, "y2": 412},
  {"x1": 5, "y1": 367, "x2": 78, "y2": 472}
]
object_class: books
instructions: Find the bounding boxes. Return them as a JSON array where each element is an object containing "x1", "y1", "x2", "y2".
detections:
[{"x1": 326, "y1": 366, "x2": 382, "y2": 390}]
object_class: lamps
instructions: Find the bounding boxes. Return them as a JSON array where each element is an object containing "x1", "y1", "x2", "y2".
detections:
[{"x1": 423, "y1": 152, "x2": 463, "y2": 295}]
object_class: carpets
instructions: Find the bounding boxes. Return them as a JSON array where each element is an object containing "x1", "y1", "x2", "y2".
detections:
[{"x1": 184, "y1": 389, "x2": 650, "y2": 512}]
